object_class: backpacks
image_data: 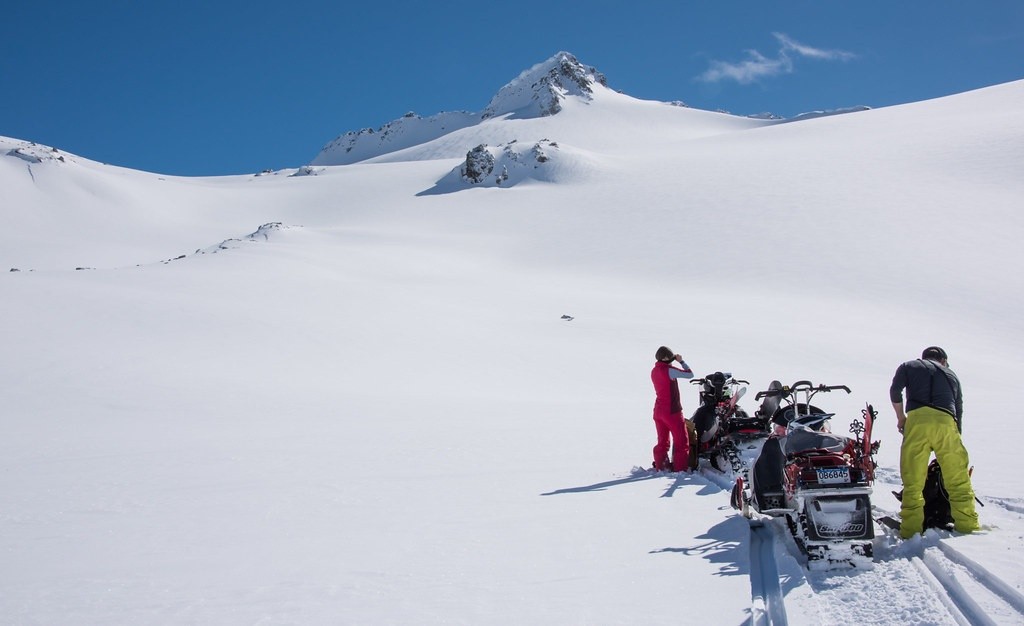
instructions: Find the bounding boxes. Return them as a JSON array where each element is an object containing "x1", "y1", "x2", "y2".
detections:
[{"x1": 892, "y1": 457, "x2": 984, "y2": 533}]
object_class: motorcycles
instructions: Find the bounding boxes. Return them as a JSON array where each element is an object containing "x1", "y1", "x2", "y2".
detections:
[
  {"x1": 688, "y1": 372, "x2": 785, "y2": 483},
  {"x1": 731, "y1": 380, "x2": 882, "y2": 573}
]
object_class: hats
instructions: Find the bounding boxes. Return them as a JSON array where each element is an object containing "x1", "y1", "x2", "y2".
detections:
[
  {"x1": 922, "y1": 346, "x2": 949, "y2": 368},
  {"x1": 655, "y1": 346, "x2": 676, "y2": 364}
]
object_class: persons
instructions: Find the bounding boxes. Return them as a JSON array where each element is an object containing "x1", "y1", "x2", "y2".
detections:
[
  {"x1": 651, "y1": 346, "x2": 693, "y2": 472},
  {"x1": 890, "y1": 346, "x2": 979, "y2": 538}
]
